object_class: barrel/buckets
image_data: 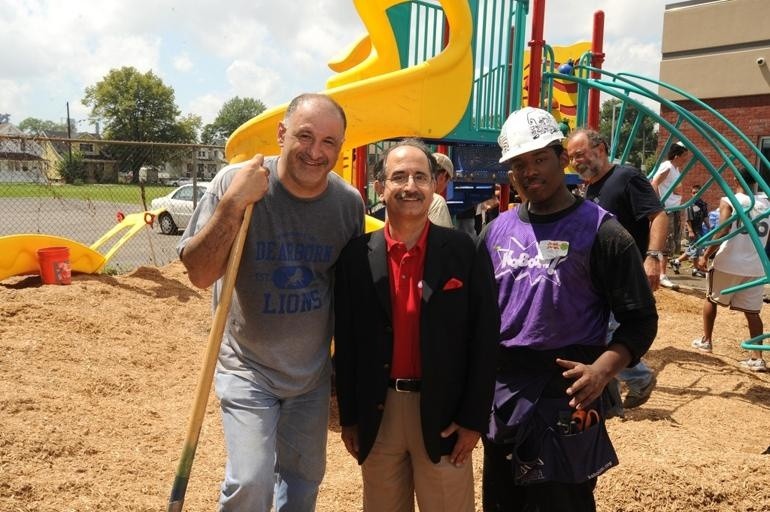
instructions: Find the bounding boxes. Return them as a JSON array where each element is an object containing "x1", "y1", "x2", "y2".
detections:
[{"x1": 36, "y1": 246, "x2": 72, "y2": 285}]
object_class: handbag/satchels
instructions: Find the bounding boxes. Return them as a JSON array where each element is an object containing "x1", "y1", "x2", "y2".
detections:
[{"x1": 508, "y1": 397, "x2": 619, "y2": 485}]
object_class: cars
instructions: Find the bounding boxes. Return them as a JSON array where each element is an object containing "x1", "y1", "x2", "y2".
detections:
[
  {"x1": 164, "y1": 176, "x2": 191, "y2": 186},
  {"x1": 150, "y1": 182, "x2": 211, "y2": 236}
]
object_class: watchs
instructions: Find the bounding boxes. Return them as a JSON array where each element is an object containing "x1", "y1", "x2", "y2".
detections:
[{"x1": 646, "y1": 251, "x2": 663, "y2": 262}]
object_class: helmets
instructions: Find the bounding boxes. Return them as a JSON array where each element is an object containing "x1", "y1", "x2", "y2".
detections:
[{"x1": 497, "y1": 107, "x2": 566, "y2": 165}]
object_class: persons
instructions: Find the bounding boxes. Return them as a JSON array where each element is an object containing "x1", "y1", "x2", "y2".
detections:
[
  {"x1": 427, "y1": 152, "x2": 455, "y2": 230},
  {"x1": 174, "y1": 93, "x2": 365, "y2": 512},
  {"x1": 691, "y1": 167, "x2": 770, "y2": 370},
  {"x1": 333, "y1": 138, "x2": 500, "y2": 512},
  {"x1": 669, "y1": 185, "x2": 712, "y2": 277},
  {"x1": 475, "y1": 107, "x2": 659, "y2": 512},
  {"x1": 565, "y1": 126, "x2": 669, "y2": 409},
  {"x1": 651, "y1": 144, "x2": 688, "y2": 288}
]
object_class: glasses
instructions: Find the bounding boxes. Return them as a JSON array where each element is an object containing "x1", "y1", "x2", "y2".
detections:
[{"x1": 388, "y1": 174, "x2": 431, "y2": 190}]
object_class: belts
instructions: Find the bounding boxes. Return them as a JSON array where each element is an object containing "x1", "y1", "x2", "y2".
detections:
[{"x1": 390, "y1": 375, "x2": 422, "y2": 393}]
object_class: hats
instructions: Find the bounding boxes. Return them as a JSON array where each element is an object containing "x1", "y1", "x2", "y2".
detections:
[{"x1": 432, "y1": 152, "x2": 455, "y2": 179}]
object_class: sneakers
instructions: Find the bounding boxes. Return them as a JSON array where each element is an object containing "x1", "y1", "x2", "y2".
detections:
[
  {"x1": 692, "y1": 338, "x2": 712, "y2": 352},
  {"x1": 739, "y1": 359, "x2": 767, "y2": 372},
  {"x1": 622, "y1": 379, "x2": 657, "y2": 410},
  {"x1": 668, "y1": 259, "x2": 682, "y2": 274},
  {"x1": 692, "y1": 270, "x2": 705, "y2": 278}
]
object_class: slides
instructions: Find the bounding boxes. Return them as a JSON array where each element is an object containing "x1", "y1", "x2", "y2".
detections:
[{"x1": 224, "y1": 0, "x2": 474, "y2": 357}]
